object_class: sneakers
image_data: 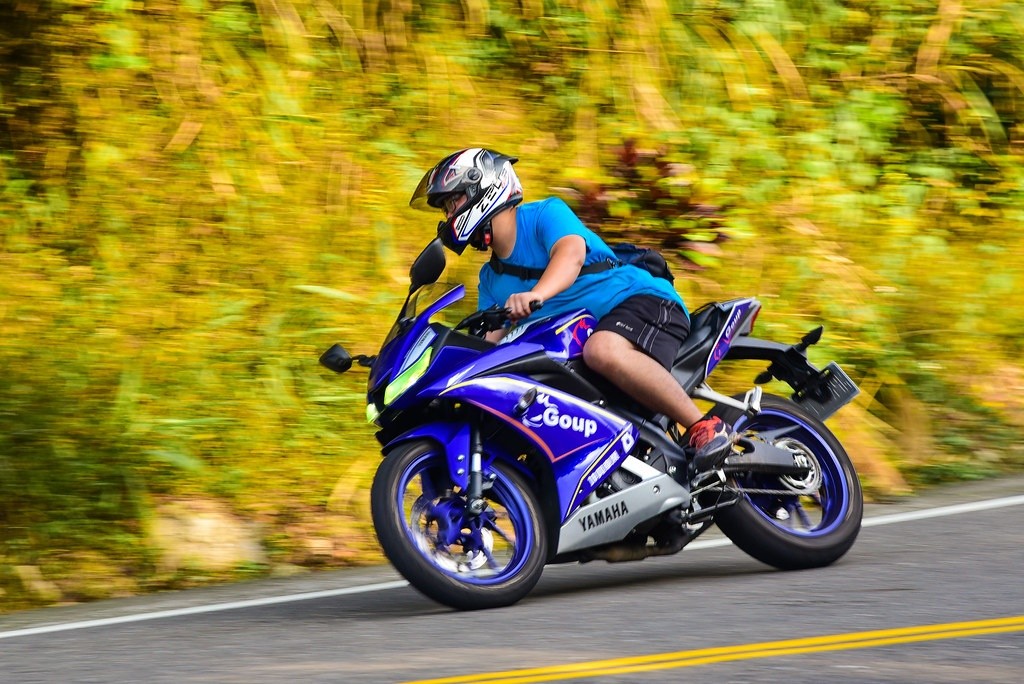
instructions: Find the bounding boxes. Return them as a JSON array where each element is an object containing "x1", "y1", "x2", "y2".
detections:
[{"x1": 688, "y1": 415, "x2": 739, "y2": 473}]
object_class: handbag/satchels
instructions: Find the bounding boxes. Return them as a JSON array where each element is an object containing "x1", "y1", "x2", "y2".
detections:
[{"x1": 609, "y1": 241, "x2": 675, "y2": 283}]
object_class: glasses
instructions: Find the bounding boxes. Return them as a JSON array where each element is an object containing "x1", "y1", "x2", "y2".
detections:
[{"x1": 440, "y1": 191, "x2": 467, "y2": 217}]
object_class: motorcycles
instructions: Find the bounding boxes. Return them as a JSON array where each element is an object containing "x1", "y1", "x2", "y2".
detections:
[{"x1": 315, "y1": 240, "x2": 865, "y2": 607}]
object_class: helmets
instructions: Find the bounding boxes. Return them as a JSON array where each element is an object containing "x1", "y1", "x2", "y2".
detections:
[{"x1": 409, "y1": 145, "x2": 523, "y2": 255}]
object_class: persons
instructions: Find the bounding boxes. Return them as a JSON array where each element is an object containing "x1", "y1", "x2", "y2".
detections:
[{"x1": 411, "y1": 147, "x2": 741, "y2": 460}]
